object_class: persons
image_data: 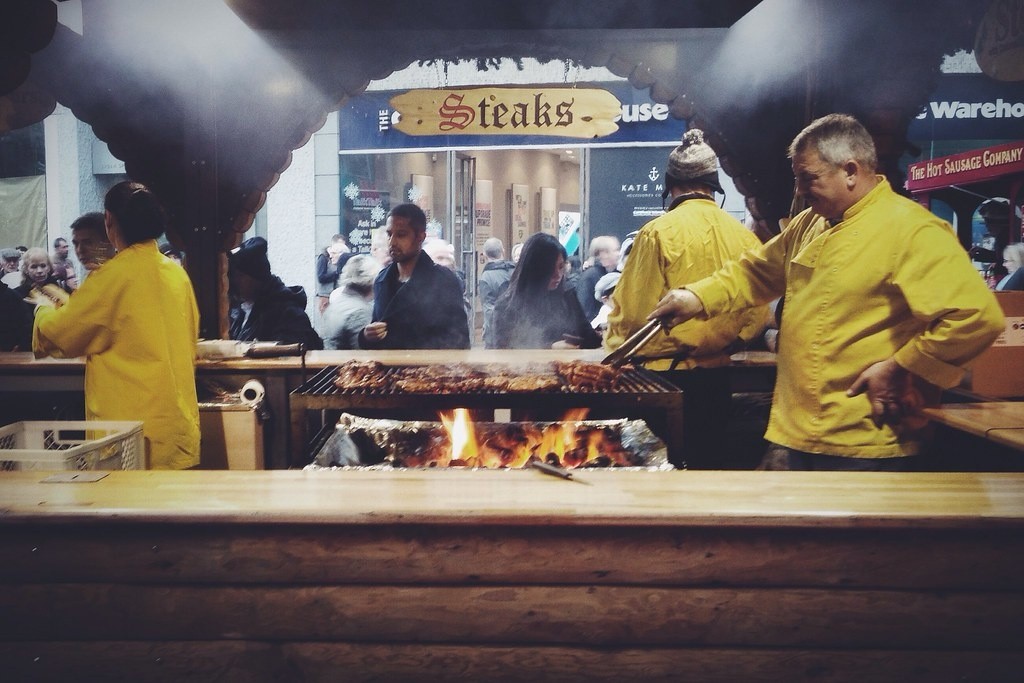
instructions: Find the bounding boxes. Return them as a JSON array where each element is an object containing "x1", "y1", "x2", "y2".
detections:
[
  {"x1": 29, "y1": 182, "x2": 203, "y2": 472},
  {"x1": 485, "y1": 234, "x2": 601, "y2": 351},
  {"x1": 994, "y1": 244, "x2": 1024, "y2": 293},
  {"x1": 479, "y1": 237, "x2": 518, "y2": 348},
  {"x1": 0, "y1": 235, "x2": 190, "y2": 353},
  {"x1": 324, "y1": 254, "x2": 380, "y2": 349},
  {"x1": 647, "y1": 113, "x2": 1006, "y2": 475},
  {"x1": 312, "y1": 230, "x2": 530, "y2": 320},
  {"x1": 419, "y1": 239, "x2": 476, "y2": 348},
  {"x1": 359, "y1": 204, "x2": 472, "y2": 349},
  {"x1": 226, "y1": 236, "x2": 325, "y2": 351},
  {"x1": 963, "y1": 199, "x2": 1024, "y2": 293},
  {"x1": 69, "y1": 212, "x2": 117, "y2": 279},
  {"x1": 600, "y1": 127, "x2": 770, "y2": 471},
  {"x1": 558, "y1": 229, "x2": 640, "y2": 349}
]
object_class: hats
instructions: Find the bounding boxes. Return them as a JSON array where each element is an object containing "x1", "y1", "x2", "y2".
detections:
[
  {"x1": 1, "y1": 247, "x2": 21, "y2": 259},
  {"x1": 232, "y1": 235, "x2": 270, "y2": 278},
  {"x1": 660, "y1": 130, "x2": 727, "y2": 209},
  {"x1": 617, "y1": 239, "x2": 637, "y2": 269},
  {"x1": 595, "y1": 273, "x2": 625, "y2": 303}
]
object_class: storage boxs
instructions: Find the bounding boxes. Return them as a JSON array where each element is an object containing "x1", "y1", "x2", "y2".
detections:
[
  {"x1": 972, "y1": 289, "x2": 1024, "y2": 401},
  {"x1": 0, "y1": 421, "x2": 147, "y2": 470}
]
